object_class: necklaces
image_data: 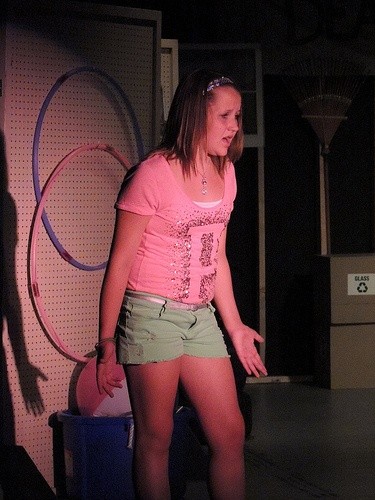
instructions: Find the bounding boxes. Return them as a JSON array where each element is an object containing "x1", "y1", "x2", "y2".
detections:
[{"x1": 196, "y1": 155, "x2": 212, "y2": 195}]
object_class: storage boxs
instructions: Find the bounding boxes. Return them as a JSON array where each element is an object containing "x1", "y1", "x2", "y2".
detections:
[
  {"x1": 325, "y1": 252, "x2": 375, "y2": 325},
  {"x1": 330, "y1": 325, "x2": 375, "y2": 388}
]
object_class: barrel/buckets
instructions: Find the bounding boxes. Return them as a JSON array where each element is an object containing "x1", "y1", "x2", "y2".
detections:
[{"x1": 49, "y1": 408, "x2": 135, "y2": 500}]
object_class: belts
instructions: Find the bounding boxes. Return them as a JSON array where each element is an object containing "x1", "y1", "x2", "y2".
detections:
[{"x1": 126, "y1": 292, "x2": 211, "y2": 311}]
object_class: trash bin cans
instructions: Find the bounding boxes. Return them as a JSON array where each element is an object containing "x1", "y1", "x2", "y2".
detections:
[{"x1": 48, "y1": 409, "x2": 180, "y2": 500}]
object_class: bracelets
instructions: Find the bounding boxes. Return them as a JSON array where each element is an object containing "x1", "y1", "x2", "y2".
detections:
[{"x1": 94, "y1": 337, "x2": 117, "y2": 350}]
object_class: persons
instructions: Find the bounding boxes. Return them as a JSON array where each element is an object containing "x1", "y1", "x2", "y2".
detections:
[{"x1": 93, "y1": 67, "x2": 268, "y2": 500}]
